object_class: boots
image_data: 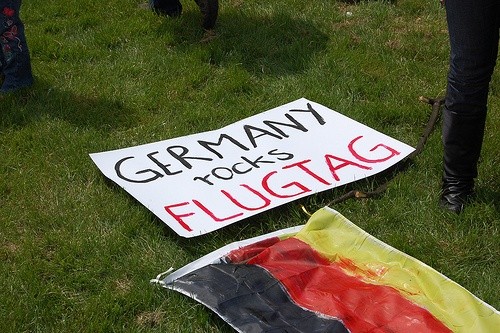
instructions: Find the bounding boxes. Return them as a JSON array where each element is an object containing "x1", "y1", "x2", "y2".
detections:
[{"x1": 438, "y1": 106, "x2": 489, "y2": 213}]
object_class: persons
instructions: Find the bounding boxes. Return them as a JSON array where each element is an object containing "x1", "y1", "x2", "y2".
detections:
[
  {"x1": 150, "y1": 0, "x2": 220, "y2": 45},
  {"x1": 0, "y1": 0, "x2": 34, "y2": 91},
  {"x1": 441, "y1": 1, "x2": 499, "y2": 214}
]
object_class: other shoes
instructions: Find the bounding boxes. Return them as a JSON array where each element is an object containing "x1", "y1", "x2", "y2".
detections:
[{"x1": 199, "y1": 1, "x2": 219, "y2": 29}]
